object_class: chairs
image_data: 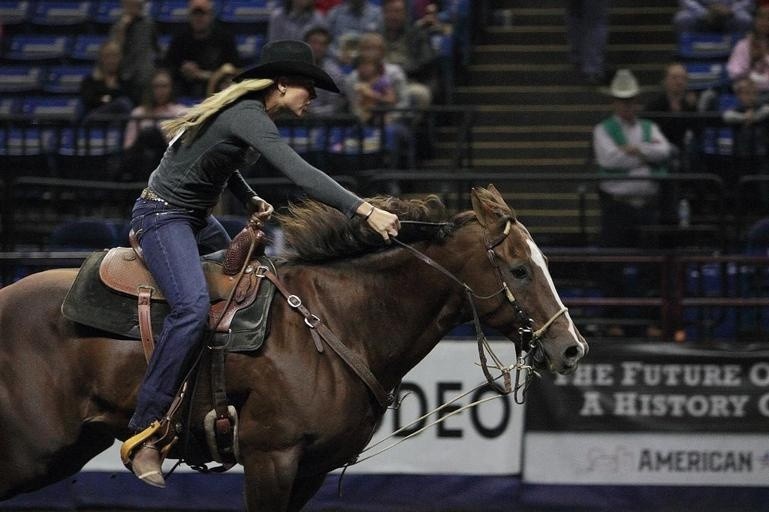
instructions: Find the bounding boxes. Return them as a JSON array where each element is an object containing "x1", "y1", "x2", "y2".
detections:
[
  {"x1": 0, "y1": 0, "x2": 490, "y2": 286},
  {"x1": 656, "y1": 1, "x2": 769, "y2": 344}
]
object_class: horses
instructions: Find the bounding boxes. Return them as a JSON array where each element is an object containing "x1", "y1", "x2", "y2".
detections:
[{"x1": 0, "y1": 184, "x2": 589, "y2": 512}]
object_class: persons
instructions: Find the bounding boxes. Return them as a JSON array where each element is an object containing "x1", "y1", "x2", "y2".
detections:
[
  {"x1": 128, "y1": 41, "x2": 402, "y2": 490},
  {"x1": 567, "y1": 2, "x2": 769, "y2": 342},
  {"x1": 79, "y1": 1, "x2": 476, "y2": 154}
]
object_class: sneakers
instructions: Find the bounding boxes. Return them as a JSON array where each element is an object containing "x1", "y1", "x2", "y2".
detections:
[{"x1": 132, "y1": 441, "x2": 166, "y2": 488}]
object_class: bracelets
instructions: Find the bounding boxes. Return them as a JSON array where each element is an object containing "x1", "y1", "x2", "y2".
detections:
[{"x1": 363, "y1": 205, "x2": 374, "y2": 221}]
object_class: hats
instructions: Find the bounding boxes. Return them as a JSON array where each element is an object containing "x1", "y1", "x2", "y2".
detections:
[
  {"x1": 610, "y1": 67, "x2": 641, "y2": 99},
  {"x1": 232, "y1": 41, "x2": 341, "y2": 94}
]
